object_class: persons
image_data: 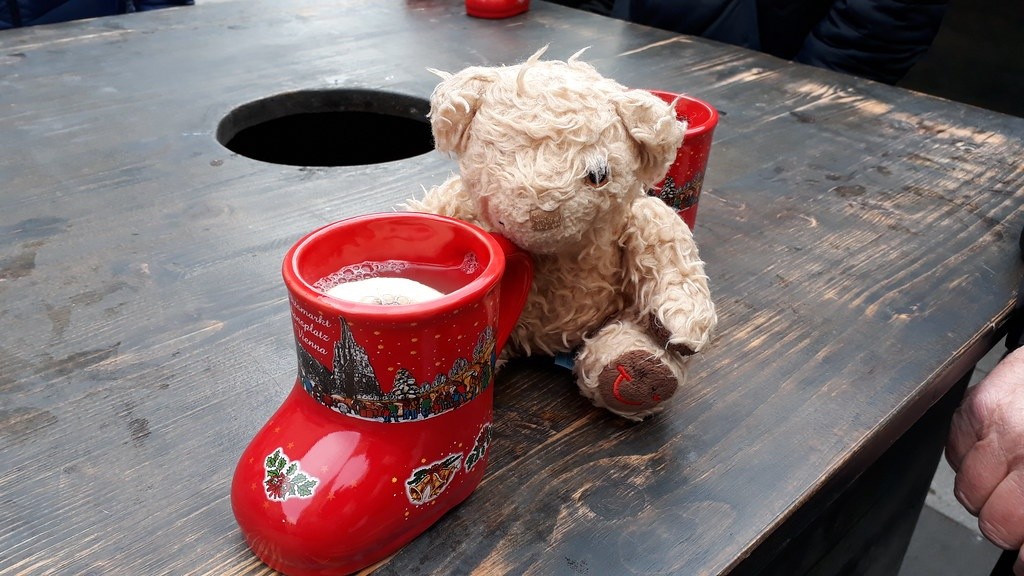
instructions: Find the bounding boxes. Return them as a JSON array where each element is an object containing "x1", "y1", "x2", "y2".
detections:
[
  {"x1": 548, "y1": 0, "x2": 946, "y2": 99},
  {"x1": 0, "y1": 0, "x2": 195, "y2": 30},
  {"x1": 944, "y1": 226, "x2": 1024, "y2": 576}
]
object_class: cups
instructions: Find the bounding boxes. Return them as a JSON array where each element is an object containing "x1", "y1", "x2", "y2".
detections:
[
  {"x1": 231, "y1": 213, "x2": 533, "y2": 576},
  {"x1": 630, "y1": 88, "x2": 718, "y2": 229},
  {"x1": 464, "y1": 0, "x2": 529, "y2": 17}
]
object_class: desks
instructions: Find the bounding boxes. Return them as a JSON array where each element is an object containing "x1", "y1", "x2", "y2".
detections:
[{"x1": 0, "y1": 0, "x2": 1024, "y2": 576}]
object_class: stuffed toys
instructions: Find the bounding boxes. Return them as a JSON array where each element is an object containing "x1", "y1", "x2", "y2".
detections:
[{"x1": 393, "y1": 52, "x2": 718, "y2": 418}]
